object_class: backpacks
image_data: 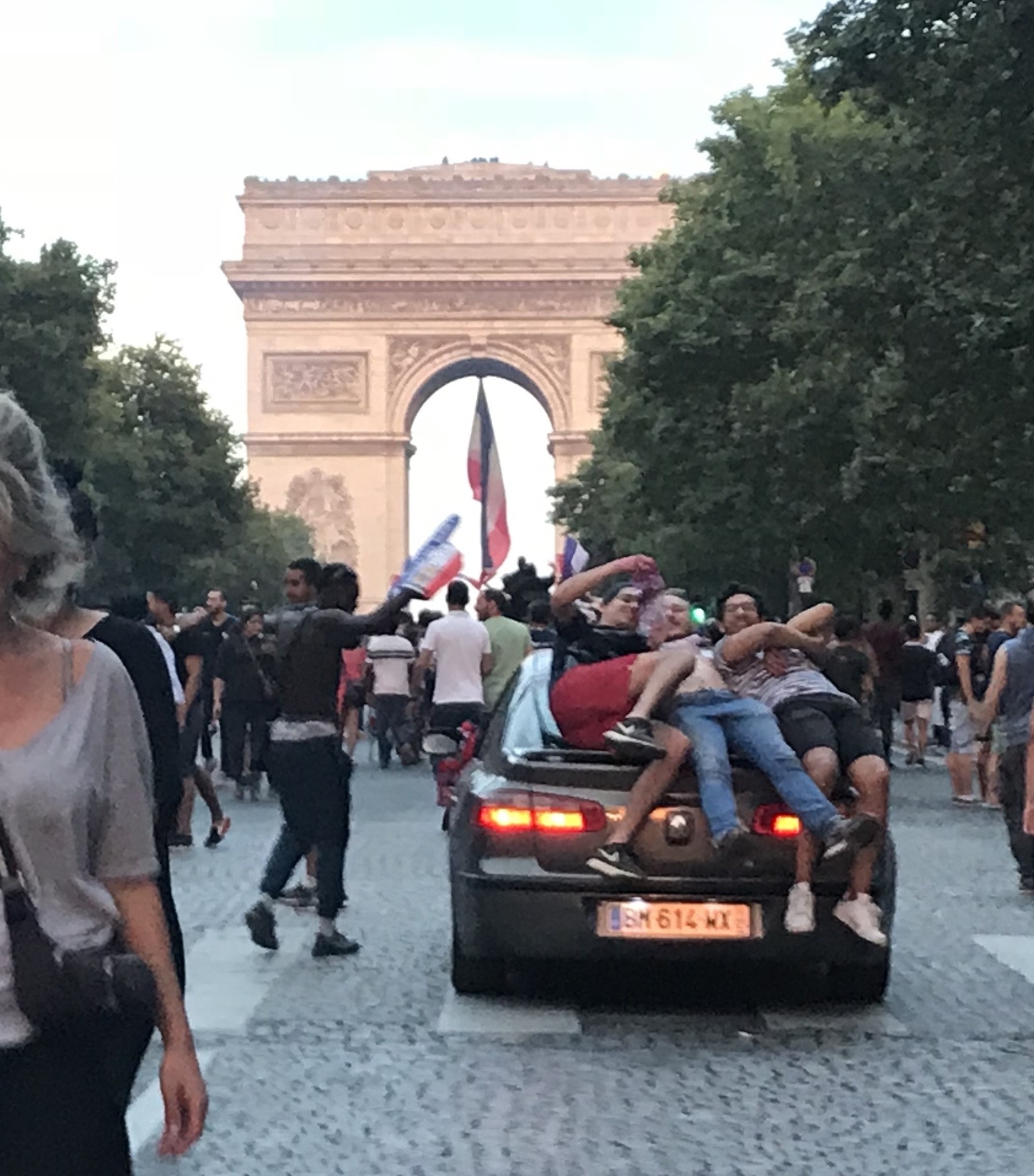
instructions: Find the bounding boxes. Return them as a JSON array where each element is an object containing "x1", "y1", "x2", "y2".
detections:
[{"x1": 931, "y1": 630, "x2": 970, "y2": 685}]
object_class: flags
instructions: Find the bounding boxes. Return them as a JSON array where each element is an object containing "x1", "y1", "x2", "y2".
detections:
[{"x1": 468, "y1": 381, "x2": 512, "y2": 585}]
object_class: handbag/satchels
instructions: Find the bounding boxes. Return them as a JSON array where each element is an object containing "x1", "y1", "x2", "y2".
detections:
[{"x1": 2, "y1": 879, "x2": 159, "y2": 1039}]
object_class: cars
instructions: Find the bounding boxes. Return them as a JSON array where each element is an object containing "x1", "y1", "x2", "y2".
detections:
[{"x1": 416, "y1": 646, "x2": 897, "y2": 1005}]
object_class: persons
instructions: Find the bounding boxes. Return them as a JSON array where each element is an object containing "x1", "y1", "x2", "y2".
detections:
[{"x1": 1, "y1": 389, "x2": 1034, "y2": 1176}]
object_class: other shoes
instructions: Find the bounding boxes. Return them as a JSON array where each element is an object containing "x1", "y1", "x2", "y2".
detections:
[
  {"x1": 904, "y1": 750, "x2": 1002, "y2": 810},
  {"x1": 311, "y1": 932, "x2": 359, "y2": 958},
  {"x1": 727, "y1": 842, "x2": 767, "y2": 880},
  {"x1": 245, "y1": 899, "x2": 279, "y2": 951},
  {"x1": 278, "y1": 880, "x2": 320, "y2": 905},
  {"x1": 206, "y1": 815, "x2": 232, "y2": 847},
  {"x1": 1018, "y1": 873, "x2": 1034, "y2": 895},
  {"x1": 166, "y1": 834, "x2": 190, "y2": 847},
  {"x1": 825, "y1": 814, "x2": 879, "y2": 862}
]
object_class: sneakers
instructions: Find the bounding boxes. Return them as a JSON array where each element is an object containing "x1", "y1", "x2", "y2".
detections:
[
  {"x1": 832, "y1": 891, "x2": 887, "y2": 944},
  {"x1": 602, "y1": 718, "x2": 668, "y2": 761},
  {"x1": 586, "y1": 846, "x2": 643, "y2": 881},
  {"x1": 785, "y1": 886, "x2": 815, "y2": 933}
]
